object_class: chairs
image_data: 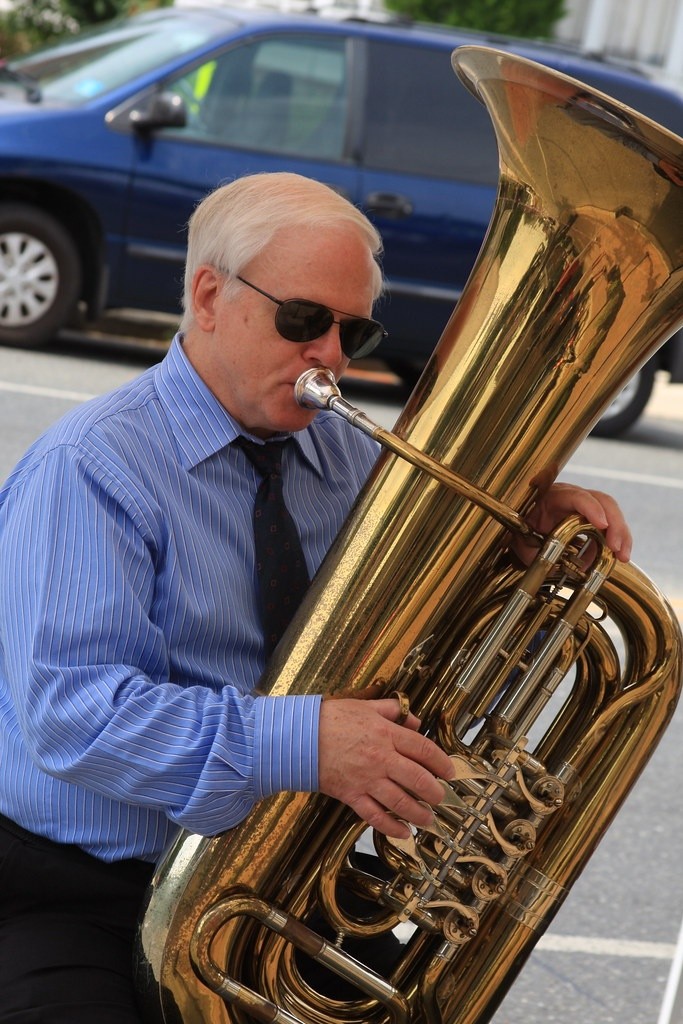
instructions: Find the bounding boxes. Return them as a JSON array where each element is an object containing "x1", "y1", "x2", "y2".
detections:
[{"x1": 225, "y1": 70, "x2": 292, "y2": 151}]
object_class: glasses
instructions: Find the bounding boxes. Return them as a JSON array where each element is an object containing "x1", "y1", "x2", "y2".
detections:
[{"x1": 237, "y1": 274, "x2": 390, "y2": 362}]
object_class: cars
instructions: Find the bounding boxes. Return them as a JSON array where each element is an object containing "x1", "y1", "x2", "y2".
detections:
[{"x1": 0, "y1": 10, "x2": 683, "y2": 438}]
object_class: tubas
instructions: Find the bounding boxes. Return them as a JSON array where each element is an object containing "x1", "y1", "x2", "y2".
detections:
[{"x1": 132, "y1": 39, "x2": 683, "y2": 1024}]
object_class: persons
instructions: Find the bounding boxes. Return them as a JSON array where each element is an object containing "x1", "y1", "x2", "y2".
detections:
[{"x1": 0, "y1": 169, "x2": 632, "y2": 1023}]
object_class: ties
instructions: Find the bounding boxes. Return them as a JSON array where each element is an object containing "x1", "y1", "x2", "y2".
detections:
[{"x1": 231, "y1": 435, "x2": 309, "y2": 666}]
